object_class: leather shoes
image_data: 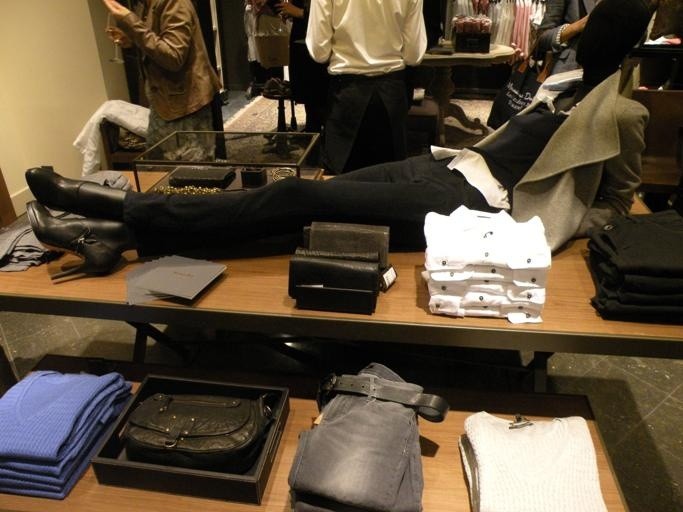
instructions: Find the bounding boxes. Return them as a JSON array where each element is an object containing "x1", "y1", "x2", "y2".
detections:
[{"x1": 25, "y1": 166, "x2": 110, "y2": 217}]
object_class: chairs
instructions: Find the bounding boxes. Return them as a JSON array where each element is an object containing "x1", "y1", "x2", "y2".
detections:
[{"x1": 100, "y1": 117, "x2": 163, "y2": 169}]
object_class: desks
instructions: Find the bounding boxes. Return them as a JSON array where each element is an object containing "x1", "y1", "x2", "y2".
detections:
[
  {"x1": 1, "y1": 168, "x2": 683, "y2": 380},
  {"x1": 1, "y1": 394, "x2": 630, "y2": 512},
  {"x1": 416, "y1": 39, "x2": 521, "y2": 147}
]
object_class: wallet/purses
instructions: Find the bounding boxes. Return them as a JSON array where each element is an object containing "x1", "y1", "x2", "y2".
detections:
[{"x1": 169, "y1": 166, "x2": 236, "y2": 188}]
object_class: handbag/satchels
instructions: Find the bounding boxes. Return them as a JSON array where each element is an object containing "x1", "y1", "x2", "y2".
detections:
[
  {"x1": 487, "y1": 33, "x2": 554, "y2": 132},
  {"x1": 118, "y1": 389, "x2": 279, "y2": 478},
  {"x1": 254, "y1": 14, "x2": 291, "y2": 69},
  {"x1": 286, "y1": 221, "x2": 391, "y2": 317}
]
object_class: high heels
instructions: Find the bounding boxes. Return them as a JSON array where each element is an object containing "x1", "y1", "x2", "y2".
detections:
[{"x1": 25, "y1": 200, "x2": 124, "y2": 280}]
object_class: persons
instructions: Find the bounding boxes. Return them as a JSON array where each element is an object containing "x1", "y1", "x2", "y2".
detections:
[
  {"x1": 25, "y1": 2, "x2": 648, "y2": 280},
  {"x1": 304, "y1": 0, "x2": 427, "y2": 172},
  {"x1": 274, "y1": 1, "x2": 329, "y2": 151},
  {"x1": 99, "y1": 1, "x2": 223, "y2": 158},
  {"x1": 533, "y1": 1, "x2": 596, "y2": 80}
]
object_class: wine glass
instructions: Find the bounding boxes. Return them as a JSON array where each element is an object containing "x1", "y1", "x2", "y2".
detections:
[
  {"x1": 271, "y1": 0, "x2": 290, "y2": 35},
  {"x1": 106, "y1": 13, "x2": 125, "y2": 64}
]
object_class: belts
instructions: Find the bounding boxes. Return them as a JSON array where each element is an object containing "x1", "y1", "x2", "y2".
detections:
[{"x1": 315, "y1": 374, "x2": 447, "y2": 424}]
